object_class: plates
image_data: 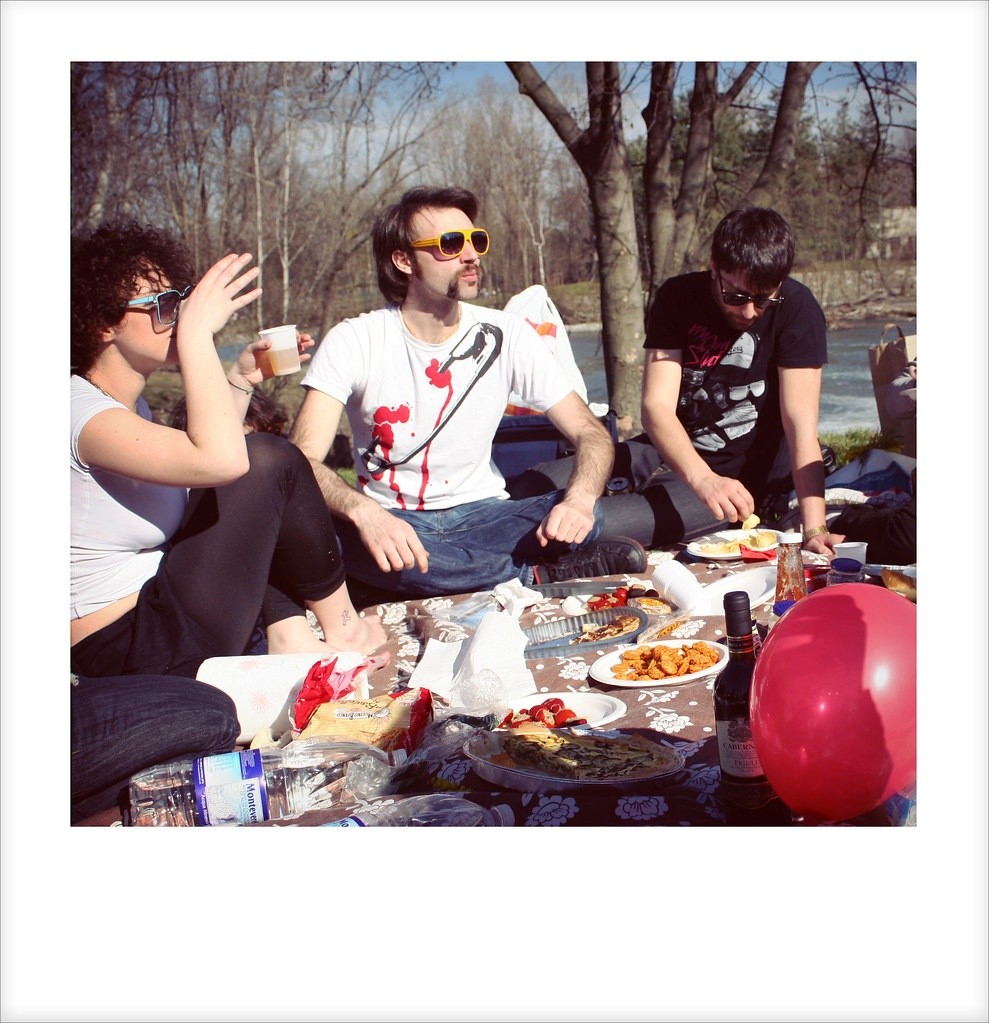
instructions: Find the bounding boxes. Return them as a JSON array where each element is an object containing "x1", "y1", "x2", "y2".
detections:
[
  {"x1": 686, "y1": 529, "x2": 784, "y2": 559},
  {"x1": 561, "y1": 580, "x2": 661, "y2": 616},
  {"x1": 491, "y1": 692, "x2": 627, "y2": 732},
  {"x1": 704, "y1": 565, "x2": 777, "y2": 610},
  {"x1": 589, "y1": 640, "x2": 730, "y2": 687}
]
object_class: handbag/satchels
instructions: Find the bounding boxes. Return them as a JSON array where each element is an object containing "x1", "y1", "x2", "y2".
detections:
[
  {"x1": 491, "y1": 408, "x2": 623, "y2": 497},
  {"x1": 867, "y1": 323, "x2": 917, "y2": 442}
]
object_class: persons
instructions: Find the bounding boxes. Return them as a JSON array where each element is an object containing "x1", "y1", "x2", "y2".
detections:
[
  {"x1": 290, "y1": 186, "x2": 648, "y2": 613},
  {"x1": 72, "y1": 210, "x2": 388, "y2": 827},
  {"x1": 164, "y1": 384, "x2": 291, "y2": 438},
  {"x1": 501, "y1": 208, "x2": 847, "y2": 555}
]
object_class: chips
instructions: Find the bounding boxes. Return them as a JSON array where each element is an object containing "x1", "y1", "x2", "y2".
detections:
[{"x1": 698, "y1": 515, "x2": 777, "y2": 553}]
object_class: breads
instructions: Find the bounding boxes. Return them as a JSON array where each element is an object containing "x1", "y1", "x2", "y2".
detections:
[{"x1": 880, "y1": 567, "x2": 915, "y2": 603}]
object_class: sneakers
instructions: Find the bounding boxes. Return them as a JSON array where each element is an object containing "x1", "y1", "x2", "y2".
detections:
[
  {"x1": 534, "y1": 534, "x2": 648, "y2": 585},
  {"x1": 345, "y1": 571, "x2": 398, "y2": 606}
]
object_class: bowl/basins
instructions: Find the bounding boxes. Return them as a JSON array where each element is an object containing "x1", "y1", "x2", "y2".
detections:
[
  {"x1": 462, "y1": 731, "x2": 685, "y2": 793},
  {"x1": 521, "y1": 607, "x2": 648, "y2": 658}
]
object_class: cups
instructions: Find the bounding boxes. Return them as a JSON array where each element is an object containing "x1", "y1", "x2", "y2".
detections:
[
  {"x1": 803, "y1": 562, "x2": 832, "y2": 594},
  {"x1": 757, "y1": 603, "x2": 773, "y2": 642},
  {"x1": 833, "y1": 542, "x2": 868, "y2": 566},
  {"x1": 258, "y1": 324, "x2": 301, "y2": 376}
]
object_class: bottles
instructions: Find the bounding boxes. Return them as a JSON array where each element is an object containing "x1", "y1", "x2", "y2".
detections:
[
  {"x1": 768, "y1": 600, "x2": 795, "y2": 633},
  {"x1": 775, "y1": 532, "x2": 806, "y2": 603},
  {"x1": 129, "y1": 741, "x2": 408, "y2": 827},
  {"x1": 714, "y1": 592, "x2": 792, "y2": 827},
  {"x1": 827, "y1": 558, "x2": 865, "y2": 586},
  {"x1": 318, "y1": 794, "x2": 515, "y2": 827}
]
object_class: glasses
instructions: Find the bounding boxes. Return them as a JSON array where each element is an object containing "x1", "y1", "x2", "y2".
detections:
[
  {"x1": 408, "y1": 228, "x2": 490, "y2": 257},
  {"x1": 121, "y1": 285, "x2": 192, "y2": 327},
  {"x1": 717, "y1": 266, "x2": 784, "y2": 309}
]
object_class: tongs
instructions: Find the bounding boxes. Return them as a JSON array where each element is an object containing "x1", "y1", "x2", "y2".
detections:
[{"x1": 526, "y1": 581, "x2": 628, "y2": 596}]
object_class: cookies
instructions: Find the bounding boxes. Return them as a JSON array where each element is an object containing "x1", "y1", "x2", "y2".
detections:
[{"x1": 610, "y1": 641, "x2": 719, "y2": 680}]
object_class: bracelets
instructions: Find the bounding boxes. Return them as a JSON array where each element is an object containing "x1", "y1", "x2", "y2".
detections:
[
  {"x1": 227, "y1": 378, "x2": 254, "y2": 395},
  {"x1": 805, "y1": 525, "x2": 829, "y2": 540}
]
